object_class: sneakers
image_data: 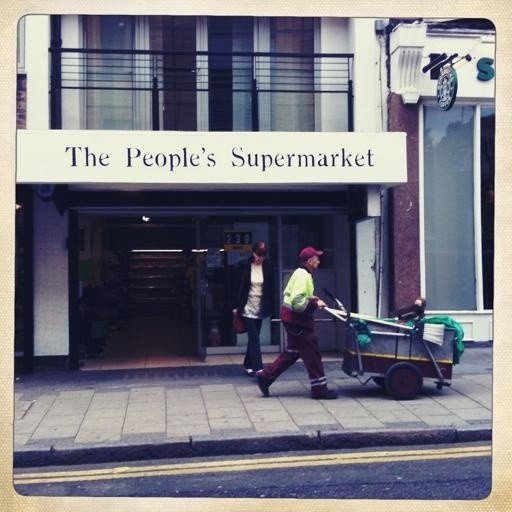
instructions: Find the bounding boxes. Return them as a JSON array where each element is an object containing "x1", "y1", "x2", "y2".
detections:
[
  {"x1": 244, "y1": 368, "x2": 257, "y2": 377},
  {"x1": 254, "y1": 369, "x2": 269, "y2": 396},
  {"x1": 310, "y1": 390, "x2": 340, "y2": 401}
]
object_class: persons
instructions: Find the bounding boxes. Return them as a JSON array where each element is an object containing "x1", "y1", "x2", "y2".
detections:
[
  {"x1": 255, "y1": 245, "x2": 340, "y2": 400},
  {"x1": 226, "y1": 239, "x2": 278, "y2": 376}
]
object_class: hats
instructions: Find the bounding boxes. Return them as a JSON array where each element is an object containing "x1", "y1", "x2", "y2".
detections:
[
  {"x1": 297, "y1": 246, "x2": 324, "y2": 260},
  {"x1": 255, "y1": 240, "x2": 267, "y2": 256}
]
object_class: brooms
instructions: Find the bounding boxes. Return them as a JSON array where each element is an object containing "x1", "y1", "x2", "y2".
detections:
[{"x1": 327, "y1": 308, "x2": 444, "y2": 346}]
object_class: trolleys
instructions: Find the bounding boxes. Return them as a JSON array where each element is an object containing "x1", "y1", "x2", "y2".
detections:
[{"x1": 319, "y1": 287, "x2": 458, "y2": 401}]
